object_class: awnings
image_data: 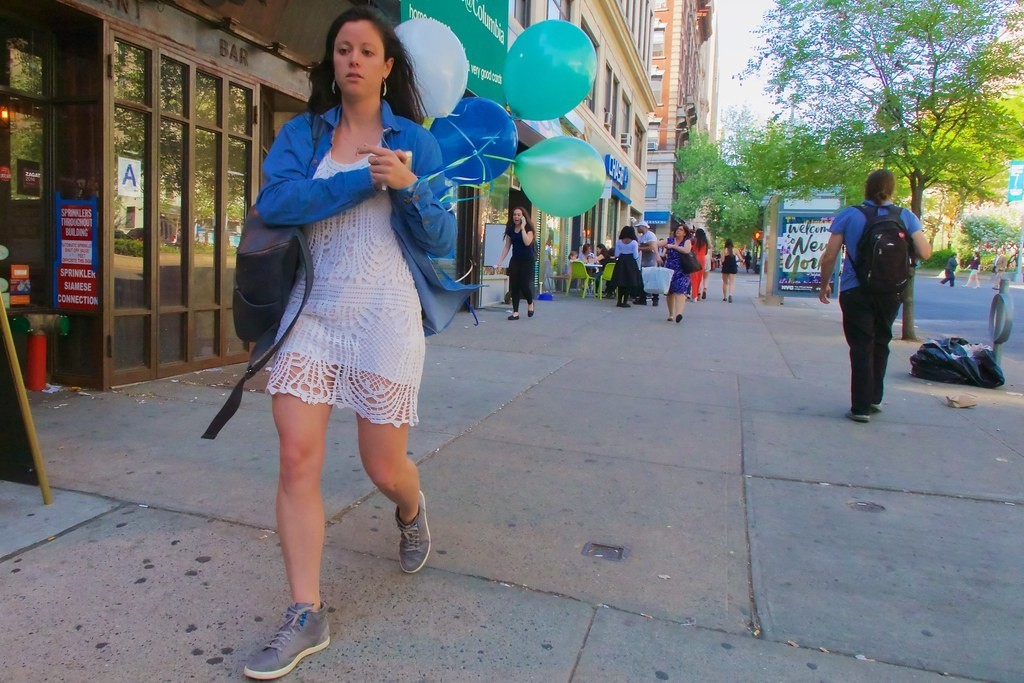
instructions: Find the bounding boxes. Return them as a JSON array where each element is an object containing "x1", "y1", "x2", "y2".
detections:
[{"x1": 644, "y1": 212, "x2": 670, "y2": 225}]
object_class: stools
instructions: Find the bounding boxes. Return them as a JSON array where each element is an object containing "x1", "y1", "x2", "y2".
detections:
[{"x1": 551, "y1": 275, "x2": 567, "y2": 293}]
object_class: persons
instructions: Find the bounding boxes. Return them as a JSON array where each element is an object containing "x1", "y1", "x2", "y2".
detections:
[
  {"x1": 244, "y1": 7, "x2": 458, "y2": 680},
  {"x1": 567, "y1": 221, "x2": 721, "y2": 308},
  {"x1": 657, "y1": 224, "x2": 692, "y2": 323},
  {"x1": 961, "y1": 251, "x2": 980, "y2": 289},
  {"x1": 745, "y1": 252, "x2": 752, "y2": 272},
  {"x1": 992, "y1": 248, "x2": 1006, "y2": 290},
  {"x1": 818, "y1": 169, "x2": 932, "y2": 422},
  {"x1": 720, "y1": 239, "x2": 744, "y2": 303},
  {"x1": 938, "y1": 252, "x2": 958, "y2": 287},
  {"x1": 495, "y1": 207, "x2": 538, "y2": 321}
]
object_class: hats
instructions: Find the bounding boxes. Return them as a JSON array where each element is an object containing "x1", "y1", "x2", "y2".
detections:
[{"x1": 634, "y1": 221, "x2": 648, "y2": 227}]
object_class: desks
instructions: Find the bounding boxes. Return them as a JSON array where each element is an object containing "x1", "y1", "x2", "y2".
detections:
[{"x1": 585, "y1": 264, "x2": 603, "y2": 298}]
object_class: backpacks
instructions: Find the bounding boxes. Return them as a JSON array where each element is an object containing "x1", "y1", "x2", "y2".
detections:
[{"x1": 844, "y1": 205, "x2": 914, "y2": 296}]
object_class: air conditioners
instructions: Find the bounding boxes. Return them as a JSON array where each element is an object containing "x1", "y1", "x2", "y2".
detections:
[
  {"x1": 605, "y1": 112, "x2": 614, "y2": 127},
  {"x1": 648, "y1": 142, "x2": 656, "y2": 151},
  {"x1": 621, "y1": 133, "x2": 632, "y2": 148}
]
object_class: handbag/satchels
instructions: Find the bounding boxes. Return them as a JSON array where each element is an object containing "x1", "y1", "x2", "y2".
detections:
[
  {"x1": 681, "y1": 240, "x2": 702, "y2": 273},
  {"x1": 232, "y1": 199, "x2": 312, "y2": 378},
  {"x1": 642, "y1": 261, "x2": 674, "y2": 295}
]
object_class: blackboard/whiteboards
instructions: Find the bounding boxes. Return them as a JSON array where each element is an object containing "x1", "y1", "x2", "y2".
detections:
[
  {"x1": 0, "y1": 294, "x2": 48, "y2": 486},
  {"x1": 482, "y1": 223, "x2": 514, "y2": 267}
]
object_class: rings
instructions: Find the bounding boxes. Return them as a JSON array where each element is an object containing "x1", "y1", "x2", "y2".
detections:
[{"x1": 375, "y1": 155, "x2": 378, "y2": 165}]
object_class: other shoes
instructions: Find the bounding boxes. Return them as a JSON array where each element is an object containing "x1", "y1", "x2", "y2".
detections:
[
  {"x1": 702, "y1": 288, "x2": 707, "y2": 300},
  {"x1": 846, "y1": 399, "x2": 881, "y2": 421},
  {"x1": 653, "y1": 298, "x2": 659, "y2": 307},
  {"x1": 938, "y1": 282, "x2": 944, "y2": 286},
  {"x1": 668, "y1": 316, "x2": 673, "y2": 321},
  {"x1": 528, "y1": 301, "x2": 534, "y2": 317},
  {"x1": 633, "y1": 299, "x2": 647, "y2": 306},
  {"x1": 962, "y1": 285, "x2": 968, "y2": 288},
  {"x1": 729, "y1": 294, "x2": 732, "y2": 304},
  {"x1": 693, "y1": 297, "x2": 698, "y2": 303},
  {"x1": 617, "y1": 302, "x2": 627, "y2": 308},
  {"x1": 723, "y1": 299, "x2": 727, "y2": 301},
  {"x1": 603, "y1": 293, "x2": 616, "y2": 299},
  {"x1": 974, "y1": 285, "x2": 979, "y2": 288},
  {"x1": 676, "y1": 314, "x2": 682, "y2": 323},
  {"x1": 508, "y1": 315, "x2": 519, "y2": 320}
]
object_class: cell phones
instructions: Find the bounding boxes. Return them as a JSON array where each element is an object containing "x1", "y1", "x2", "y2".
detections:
[{"x1": 520, "y1": 217, "x2": 524, "y2": 223}]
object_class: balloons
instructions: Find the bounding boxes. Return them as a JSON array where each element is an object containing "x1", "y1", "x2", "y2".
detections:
[
  {"x1": 429, "y1": 96, "x2": 519, "y2": 185},
  {"x1": 393, "y1": 17, "x2": 468, "y2": 117},
  {"x1": 503, "y1": 18, "x2": 597, "y2": 121},
  {"x1": 515, "y1": 135, "x2": 606, "y2": 218}
]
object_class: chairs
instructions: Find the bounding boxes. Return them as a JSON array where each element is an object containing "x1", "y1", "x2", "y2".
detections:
[
  {"x1": 565, "y1": 261, "x2": 596, "y2": 299},
  {"x1": 598, "y1": 263, "x2": 624, "y2": 301}
]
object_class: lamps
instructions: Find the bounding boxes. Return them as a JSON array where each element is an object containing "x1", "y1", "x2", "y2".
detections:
[
  {"x1": 265, "y1": 41, "x2": 287, "y2": 51},
  {"x1": 215, "y1": 16, "x2": 240, "y2": 31}
]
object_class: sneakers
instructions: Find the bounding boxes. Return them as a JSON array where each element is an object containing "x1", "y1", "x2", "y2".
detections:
[
  {"x1": 396, "y1": 487, "x2": 430, "y2": 573},
  {"x1": 244, "y1": 602, "x2": 329, "y2": 679}
]
